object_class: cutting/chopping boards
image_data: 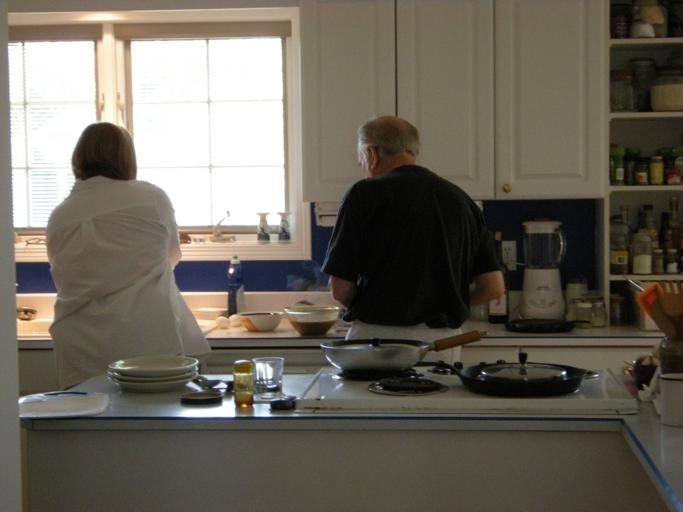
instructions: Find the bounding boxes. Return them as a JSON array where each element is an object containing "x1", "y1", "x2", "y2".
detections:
[{"x1": 17, "y1": 388, "x2": 111, "y2": 420}]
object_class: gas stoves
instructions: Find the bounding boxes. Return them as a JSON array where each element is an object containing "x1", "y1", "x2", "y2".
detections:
[{"x1": 296, "y1": 362, "x2": 638, "y2": 413}]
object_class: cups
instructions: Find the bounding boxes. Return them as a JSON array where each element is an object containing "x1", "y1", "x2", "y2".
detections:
[
  {"x1": 659, "y1": 372, "x2": 682, "y2": 427},
  {"x1": 253, "y1": 356, "x2": 284, "y2": 396}
]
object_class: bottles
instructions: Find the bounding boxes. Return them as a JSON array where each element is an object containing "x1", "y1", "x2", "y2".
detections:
[
  {"x1": 232, "y1": 359, "x2": 253, "y2": 407},
  {"x1": 226, "y1": 254, "x2": 246, "y2": 314},
  {"x1": 576, "y1": 295, "x2": 608, "y2": 329},
  {"x1": 254, "y1": 210, "x2": 290, "y2": 243},
  {"x1": 608, "y1": 138, "x2": 681, "y2": 185},
  {"x1": 609, "y1": 202, "x2": 681, "y2": 274},
  {"x1": 487, "y1": 230, "x2": 511, "y2": 325}
]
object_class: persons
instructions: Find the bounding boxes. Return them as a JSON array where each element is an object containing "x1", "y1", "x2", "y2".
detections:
[
  {"x1": 320, "y1": 115, "x2": 506, "y2": 366},
  {"x1": 45, "y1": 122, "x2": 212, "y2": 390}
]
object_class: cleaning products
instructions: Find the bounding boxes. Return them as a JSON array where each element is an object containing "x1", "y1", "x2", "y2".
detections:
[{"x1": 226, "y1": 254, "x2": 243, "y2": 317}]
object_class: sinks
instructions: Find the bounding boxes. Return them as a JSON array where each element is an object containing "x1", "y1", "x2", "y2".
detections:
[{"x1": 16, "y1": 318, "x2": 216, "y2": 342}]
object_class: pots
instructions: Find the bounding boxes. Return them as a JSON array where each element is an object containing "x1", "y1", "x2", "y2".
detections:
[
  {"x1": 447, "y1": 351, "x2": 600, "y2": 398},
  {"x1": 318, "y1": 328, "x2": 485, "y2": 372}
]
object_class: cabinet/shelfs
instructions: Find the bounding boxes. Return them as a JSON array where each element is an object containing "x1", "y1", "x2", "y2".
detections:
[
  {"x1": 493, "y1": 0, "x2": 610, "y2": 198},
  {"x1": 298, "y1": 0, "x2": 493, "y2": 204},
  {"x1": 605, "y1": 0, "x2": 683, "y2": 326}
]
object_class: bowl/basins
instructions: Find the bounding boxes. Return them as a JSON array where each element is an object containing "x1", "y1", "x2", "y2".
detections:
[
  {"x1": 236, "y1": 310, "x2": 286, "y2": 331},
  {"x1": 282, "y1": 304, "x2": 340, "y2": 335}
]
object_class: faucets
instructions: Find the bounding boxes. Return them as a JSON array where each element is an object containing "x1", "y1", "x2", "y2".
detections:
[{"x1": 16, "y1": 306, "x2": 37, "y2": 320}]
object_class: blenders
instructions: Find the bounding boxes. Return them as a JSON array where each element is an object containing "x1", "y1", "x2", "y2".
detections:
[{"x1": 520, "y1": 221, "x2": 568, "y2": 320}]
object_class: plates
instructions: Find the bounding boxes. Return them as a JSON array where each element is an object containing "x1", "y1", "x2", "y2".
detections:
[
  {"x1": 107, "y1": 353, "x2": 198, "y2": 393},
  {"x1": 506, "y1": 320, "x2": 575, "y2": 333}
]
